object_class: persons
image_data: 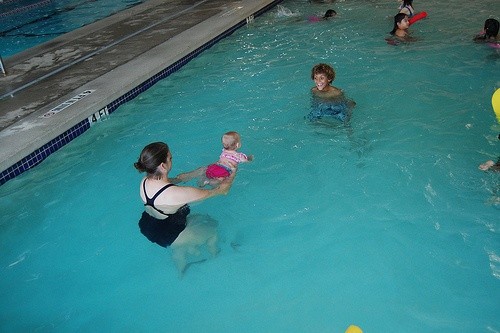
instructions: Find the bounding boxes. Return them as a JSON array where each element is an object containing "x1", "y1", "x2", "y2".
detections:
[
  {"x1": 391, "y1": 12, "x2": 409, "y2": 36},
  {"x1": 325, "y1": 9, "x2": 337, "y2": 17},
  {"x1": 223, "y1": 132, "x2": 246, "y2": 158},
  {"x1": 311, "y1": 63, "x2": 337, "y2": 91},
  {"x1": 134, "y1": 142, "x2": 209, "y2": 205},
  {"x1": 475, "y1": 18, "x2": 500, "y2": 41},
  {"x1": 398, "y1": 0, "x2": 414, "y2": 14}
]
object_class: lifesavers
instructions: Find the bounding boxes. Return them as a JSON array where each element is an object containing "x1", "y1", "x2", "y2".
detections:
[{"x1": 409, "y1": 11, "x2": 427, "y2": 27}]
